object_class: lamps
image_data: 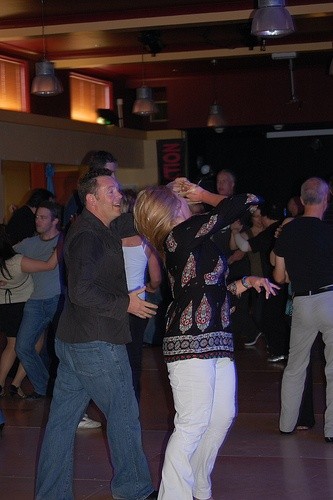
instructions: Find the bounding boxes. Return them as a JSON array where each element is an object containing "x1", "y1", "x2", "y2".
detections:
[
  {"x1": 132, "y1": 48, "x2": 159, "y2": 115},
  {"x1": 30, "y1": 0, "x2": 64, "y2": 95},
  {"x1": 206, "y1": 60, "x2": 227, "y2": 127},
  {"x1": 250, "y1": 0, "x2": 295, "y2": 39}
]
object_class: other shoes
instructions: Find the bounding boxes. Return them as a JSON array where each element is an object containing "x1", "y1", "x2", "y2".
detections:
[
  {"x1": 243, "y1": 331, "x2": 262, "y2": 346},
  {"x1": 266, "y1": 353, "x2": 284, "y2": 363},
  {"x1": 75, "y1": 414, "x2": 101, "y2": 430},
  {"x1": 296, "y1": 418, "x2": 316, "y2": 430},
  {"x1": 26, "y1": 391, "x2": 43, "y2": 400}
]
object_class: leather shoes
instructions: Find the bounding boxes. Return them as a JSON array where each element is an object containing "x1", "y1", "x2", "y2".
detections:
[{"x1": 324, "y1": 437, "x2": 333, "y2": 441}]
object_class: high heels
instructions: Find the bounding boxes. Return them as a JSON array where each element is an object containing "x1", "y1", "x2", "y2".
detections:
[
  {"x1": 0, "y1": 385, "x2": 7, "y2": 399},
  {"x1": 8, "y1": 384, "x2": 28, "y2": 400}
]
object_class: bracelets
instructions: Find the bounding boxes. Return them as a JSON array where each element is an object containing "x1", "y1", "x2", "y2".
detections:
[{"x1": 241, "y1": 276, "x2": 250, "y2": 289}]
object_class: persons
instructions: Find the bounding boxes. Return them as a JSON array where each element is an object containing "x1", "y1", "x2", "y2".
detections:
[
  {"x1": 34, "y1": 168, "x2": 189, "y2": 500},
  {"x1": 57, "y1": 150, "x2": 117, "y2": 238},
  {"x1": 229, "y1": 210, "x2": 264, "y2": 328},
  {"x1": 232, "y1": 205, "x2": 288, "y2": 361},
  {"x1": 119, "y1": 189, "x2": 162, "y2": 402},
  {"x1": 217, "y1": 169, "x2": 264, "y2": 205},
  {"x1": 0, "y1": 224, "x2": 58, "y2": 398},
  {"x1": 270, "y1": 217, "x2": 325, "y2": 430},
  {"x1": 12, "y1": 202, "x2": 66, "y2": 401},
  {"x1": 273, "y1": 177, "x2": 333, "y2": 443},
  {"x1": 133, "y1": 184, "x2": 281, "y2": 500},
  {"x1": 7, "y1": 188, "x2": 55, "y2": 237}
]
